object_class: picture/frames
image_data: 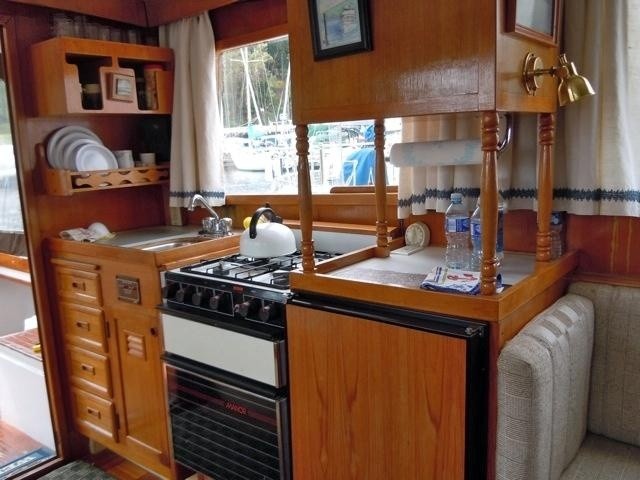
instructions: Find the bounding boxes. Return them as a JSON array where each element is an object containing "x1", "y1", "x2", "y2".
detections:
[
  {"x1": 504, "y1": 0, "x2": 564, "y2": 50},
  {"x1": 306, "y1": 0, "x2": 375, "y2": 63}
]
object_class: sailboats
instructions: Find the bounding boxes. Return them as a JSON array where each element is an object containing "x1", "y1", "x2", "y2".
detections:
[{"x1": 220, "y1": 44, "x2": 401, "y2": 188}]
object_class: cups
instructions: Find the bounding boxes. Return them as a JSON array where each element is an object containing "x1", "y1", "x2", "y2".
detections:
[
  {"x1": 112, "y1": 149, "x2": 156, "y2": 168},
  {"x1": 50, "y1": 13, "x2": 141, "y2": 46}
]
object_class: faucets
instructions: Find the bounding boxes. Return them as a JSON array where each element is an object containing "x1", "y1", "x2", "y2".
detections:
[{"x1": 187, "y1": 194, "x2": 234, "y2": 238}]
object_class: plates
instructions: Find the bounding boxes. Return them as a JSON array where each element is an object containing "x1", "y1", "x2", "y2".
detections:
[{"x1": 47, "y1": 126, "x2": 118, "y2": 188}]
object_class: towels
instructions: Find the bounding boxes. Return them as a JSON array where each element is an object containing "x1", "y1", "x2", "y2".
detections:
[
  {"x1": 420, "y1": 264, "x2": 504, "y2": 293},
  {"x1": 58, "y1": 227, "x2": 119, "y2": 242}
]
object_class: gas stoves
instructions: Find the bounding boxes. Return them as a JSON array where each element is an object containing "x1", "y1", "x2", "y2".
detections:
[{"x1": 157, "y1": 250, "x2": 345, "y2": 340}]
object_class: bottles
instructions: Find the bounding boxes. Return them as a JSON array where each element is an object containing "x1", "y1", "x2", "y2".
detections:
[
  {"x1": 549, "y1": 196, "x2": 566, "y2": 258},
  {"x1": 444, "y1": 192, "x2": 505, "y2": 271},
  {"x1": 86, "y1": 63, "x2": 163, "y2": 110}
]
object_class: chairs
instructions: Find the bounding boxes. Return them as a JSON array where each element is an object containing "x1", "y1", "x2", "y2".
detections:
[{"x1": 494, "y1": 270, "x2": 639, "y2": 479}]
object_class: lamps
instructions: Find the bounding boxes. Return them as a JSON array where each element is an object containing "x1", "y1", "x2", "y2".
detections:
[{"x1": 523, "y1": 50, "x2": 597, "y2": 107}]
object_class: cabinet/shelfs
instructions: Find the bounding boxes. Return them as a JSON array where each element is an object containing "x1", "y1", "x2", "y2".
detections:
[
  {"x1": 284, "y1": 0, "x2": 579, "y2": 478},
  {"x1": 47, "y1": 244, "x2": 240, "y2": 480},
  {"x1": 31, "y1": 35, "x2": 178, "y2": 115}
]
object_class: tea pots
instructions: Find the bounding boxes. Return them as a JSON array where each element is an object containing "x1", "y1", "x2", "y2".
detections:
[{"x1": 239, "y1": 207, "x2": 297, "y2": 258}]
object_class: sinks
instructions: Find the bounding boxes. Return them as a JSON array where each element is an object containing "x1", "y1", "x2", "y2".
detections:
[{"x1": 125, "y1": 232, "x2": 216, "y2": 253}]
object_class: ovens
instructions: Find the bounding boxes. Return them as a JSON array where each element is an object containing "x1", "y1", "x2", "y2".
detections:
[{"x1": 154, "y1": 304, "x2": 293, "y2": 480}]
